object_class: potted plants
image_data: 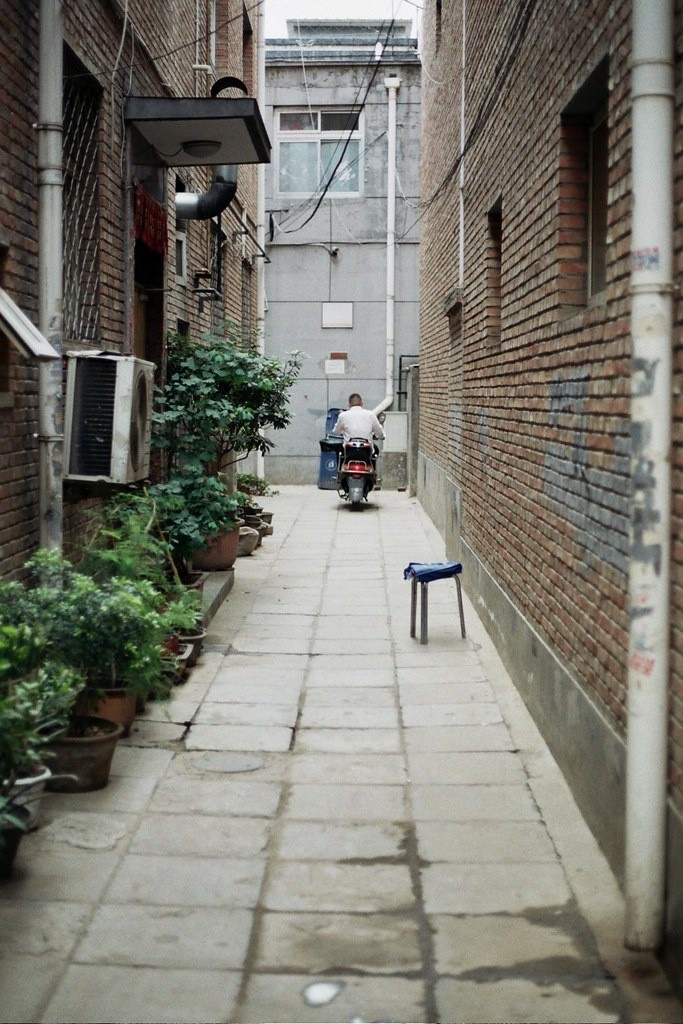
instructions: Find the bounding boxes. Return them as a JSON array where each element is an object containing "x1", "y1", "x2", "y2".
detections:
[
  {"x1": 237, "y1": 473, "x2": 280, "y2": 514},
  {"x1": 166, "y1": 600, "x2": 208, "y2": 667},
  {"x1": 144, "y1": 316, "x2": 308, "y2": 572},
  {"x1": 149, "y1": 463, "x2": 251, "y2": 604},
  {"x1": 24, "y1": 590, "x2": 159, "y2": 793},
  {"x1": 56, "y1": 583, "x2": 163, "y2": 738},
  {"x1": 0, "y1": 621, "x2": 52, "y2": 831}
]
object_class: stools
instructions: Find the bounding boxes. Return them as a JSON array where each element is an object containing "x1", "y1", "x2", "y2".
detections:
[{"x1": 403, "y1": 562, "x2": 466, "y2": 645}]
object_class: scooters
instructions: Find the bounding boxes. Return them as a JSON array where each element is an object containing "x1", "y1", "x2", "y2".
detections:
[{"x1": 337, "y1": 409, "x2": 388, "y2": 511}]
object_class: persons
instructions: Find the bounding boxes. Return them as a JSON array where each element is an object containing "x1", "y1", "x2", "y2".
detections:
[{"x1": 332, "y1": 393, "x2": 384, "y2": 470}]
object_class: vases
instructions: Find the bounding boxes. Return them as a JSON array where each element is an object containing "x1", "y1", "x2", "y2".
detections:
[
  {"x1": 259, "y1": 512, "x2": 273, "y2": 524},
  {"x1": 238, "y1": 515, "x2": 263, "y2": 528}
]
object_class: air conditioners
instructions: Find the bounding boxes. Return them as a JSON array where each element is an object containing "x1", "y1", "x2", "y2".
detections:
[{"x1": 61, "y1": 350, "x2": 155, "y2": 484}]
